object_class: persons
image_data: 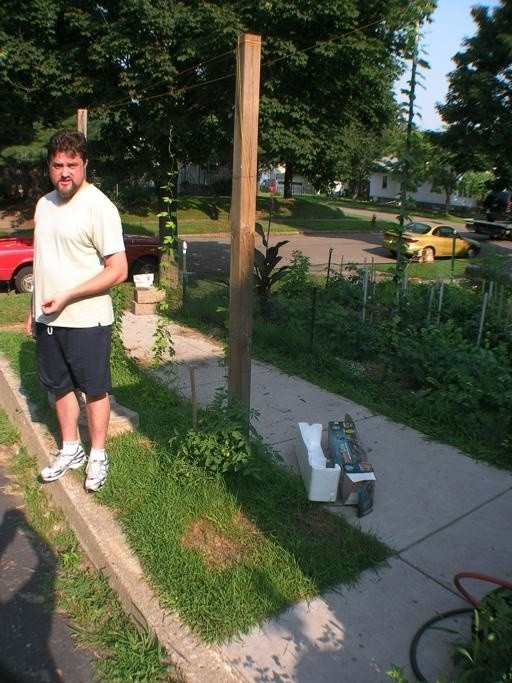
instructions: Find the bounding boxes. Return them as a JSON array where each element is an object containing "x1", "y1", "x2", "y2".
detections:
[{"x1": 26, "y1": 127, "x2": 128, "y2": 494}]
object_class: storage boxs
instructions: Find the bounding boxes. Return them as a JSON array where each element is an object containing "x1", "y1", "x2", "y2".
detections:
[
  {"x1": 130, "y1": 301, "x2": 158, "y2": 315},
  {"x1": 136, "y1": 286, "x2": 167, "y2": 303},
  {"x1": 328, "y1": 412, "x2": 377, "y2": 518}
]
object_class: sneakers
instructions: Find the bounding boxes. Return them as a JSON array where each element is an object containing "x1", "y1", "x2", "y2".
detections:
[
  {"x1": 41, "y1": 445, "x2": 86, "y2": 482},
  {"x1": 84, "y1": 449, "x2": 109, "y2": 492}
]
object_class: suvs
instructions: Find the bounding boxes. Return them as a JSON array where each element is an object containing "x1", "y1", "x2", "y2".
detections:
[{"x1": 480, "y1": 185, "x2": 511, "y2": 224}]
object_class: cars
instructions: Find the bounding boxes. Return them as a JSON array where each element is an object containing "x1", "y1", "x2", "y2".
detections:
[
  {"x1": 0, "y1": 226, "x2": 173, "y2": 293},
  {"x1": 382, "y1": 219, "x2": 482, "y2": 264}
]
